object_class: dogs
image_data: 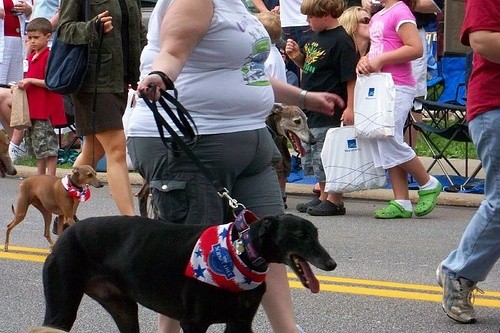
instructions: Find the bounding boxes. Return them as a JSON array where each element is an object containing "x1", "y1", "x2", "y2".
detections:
[
  {"x1": 42, "y1": 212, "x2": 338, "y2": 333},
  {"x1": 2, "y1": 164, "x2": 105, "y2": 252},
  {"x1": 264, "y1": 102, "x2": 318, "y2": 177}
]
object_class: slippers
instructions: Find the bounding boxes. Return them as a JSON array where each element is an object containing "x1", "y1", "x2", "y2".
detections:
[
  {"x1": 374, "y1": 200, "x2": 413, "y2": 219},
  {"x1": 415, "y1": 182, "x2": 442, "y2": 216}
]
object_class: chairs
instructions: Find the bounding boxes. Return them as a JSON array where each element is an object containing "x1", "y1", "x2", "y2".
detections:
[{"x1": 407, "y1": 53, "x2": 483, "y2": 192}]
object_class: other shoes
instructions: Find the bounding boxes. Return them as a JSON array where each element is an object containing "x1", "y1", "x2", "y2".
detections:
[{"x1": 53, "y1": 214, "x2": 81, "y2": 234}]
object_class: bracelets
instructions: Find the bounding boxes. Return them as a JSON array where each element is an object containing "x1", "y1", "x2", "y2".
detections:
[{"x1": 298, "y1": 90, "x2": 307, "y2": 109}]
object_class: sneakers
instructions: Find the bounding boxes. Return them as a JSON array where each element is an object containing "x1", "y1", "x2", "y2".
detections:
[{"x1": 436, "y1": 263, "x2": 484, "y2": 324}]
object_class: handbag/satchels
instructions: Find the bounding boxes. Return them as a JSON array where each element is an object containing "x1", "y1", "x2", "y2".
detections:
[
  {"x1": 320, "y1": 121, "x2": 388, "y2": 192},
  {"x1": 45, "y1": 0, "x2": 91, "y2": 94},
  {"x1": 354, "y1": 69, "x2": 395, "y2": 138},
  {"x1": 10, "y1": 85, "x2": 32, "y2": 130}
]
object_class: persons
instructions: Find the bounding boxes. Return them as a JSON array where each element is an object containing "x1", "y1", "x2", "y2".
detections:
[
  {"x1": 126, "y1": 0, "x2": 344, "y2": 333},
  {"x1": 10, "y1": 18, "x2": 67, "y2": 177},
  {"x1": 0, "y1": 0, "x2": 81, "y2": 163},
  {"x1": 52, "y1": 0, "x2": 148, "y2": 236},
  {"x1": 244, "y1": 0, "x2": 442, "y2": 218},
  {"x1": 435, "y1": 0, "x2": 500, "y2": 324}
]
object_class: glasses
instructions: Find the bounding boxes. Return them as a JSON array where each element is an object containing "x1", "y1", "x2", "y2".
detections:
[{"x1": 358, "y1": 17, "x2": 370, "y2": 24}]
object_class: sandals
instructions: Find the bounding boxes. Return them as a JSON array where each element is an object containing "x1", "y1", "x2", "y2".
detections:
[
  {"x1": 307, "y1": 200, "x2": 345, "y2": 215},
  {"x1": 296, "y1": 197, "x2": 321, "y2": 212}
]
